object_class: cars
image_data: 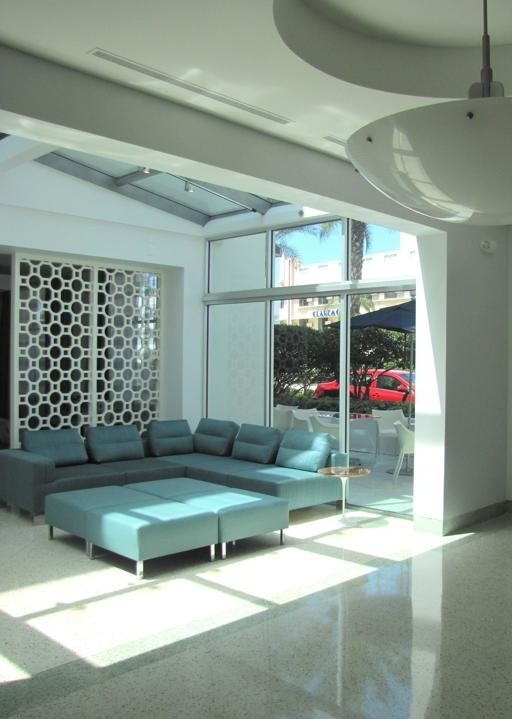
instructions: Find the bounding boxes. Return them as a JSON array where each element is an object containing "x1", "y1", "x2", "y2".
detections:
[{"x1": 313, "y1": 367, "x2": 414, "y2": 404}]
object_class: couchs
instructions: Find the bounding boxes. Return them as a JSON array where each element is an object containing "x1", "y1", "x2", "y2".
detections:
[{"x1": 0, "y1": 437, "x2": 347, "y2": 526}]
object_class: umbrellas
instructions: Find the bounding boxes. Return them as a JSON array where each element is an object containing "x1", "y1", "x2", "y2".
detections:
[{"x1": 323, "y1": 299, "x2": 416, "y2": 472}]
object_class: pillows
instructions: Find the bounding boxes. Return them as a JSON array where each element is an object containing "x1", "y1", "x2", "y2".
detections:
[
  {"x1": 192, "y1": 419, "x2": 240, "y2": 456},
  {"x1": 232, "y1": 423, "x2": 282, "y2": 464},
  {"x1": 147, "y1": 419, "x2": 194, "y2": 457},
  {"x1": 85, "y1": 425, "x2": 144, "y2": 464},
  {"x1": 20, "y1": 429, "x2": 89, "y2": 468}
]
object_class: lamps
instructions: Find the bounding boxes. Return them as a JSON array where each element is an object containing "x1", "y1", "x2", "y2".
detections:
[
  {"x1": 343, "y1": 0, "x2": 512, "y2": 228},
  {"x1": 184, "y1": 181, "x2": 195, "y2": 193},
  {"x1": 143, "y1": 168, "x2": 150, "y2": 174}
]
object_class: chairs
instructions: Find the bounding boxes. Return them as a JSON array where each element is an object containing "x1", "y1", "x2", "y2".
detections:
[{"x1": 274, "y1": 404, "x2": 414, "y2": 481}]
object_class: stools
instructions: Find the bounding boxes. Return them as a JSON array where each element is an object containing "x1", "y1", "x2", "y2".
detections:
[
  {"x1": 123, "y1": 477, "x2": 289, "y2": 559},
  {"x1": 44, "y1": 486, "x2": 221, "y2": 581}
]
